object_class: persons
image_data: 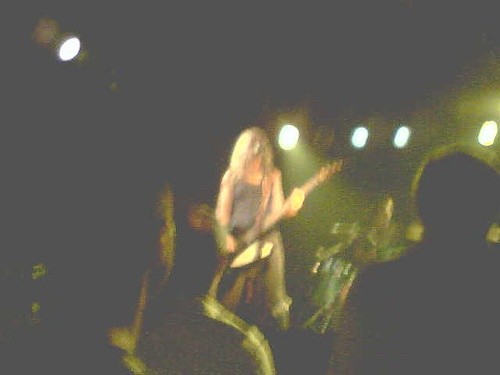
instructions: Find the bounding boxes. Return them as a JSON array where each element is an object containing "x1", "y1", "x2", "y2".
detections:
[
  {"x1": 5, "y1": 168, "x2": 180, "y2": 375},
  {"x1": 137, "y1": 196, "x2": 277, "y2": 375},
  {"x1": 327, "y1": 148, "x2": 500, "y2": 375},
  {"x1": 211, "y1": 125, "x2": 305, "y2": 329}
]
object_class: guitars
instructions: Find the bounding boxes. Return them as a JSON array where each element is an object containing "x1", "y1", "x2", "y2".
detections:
[{"x1": 216, "y1": 158, "x2": 343, "y2": 271}]
